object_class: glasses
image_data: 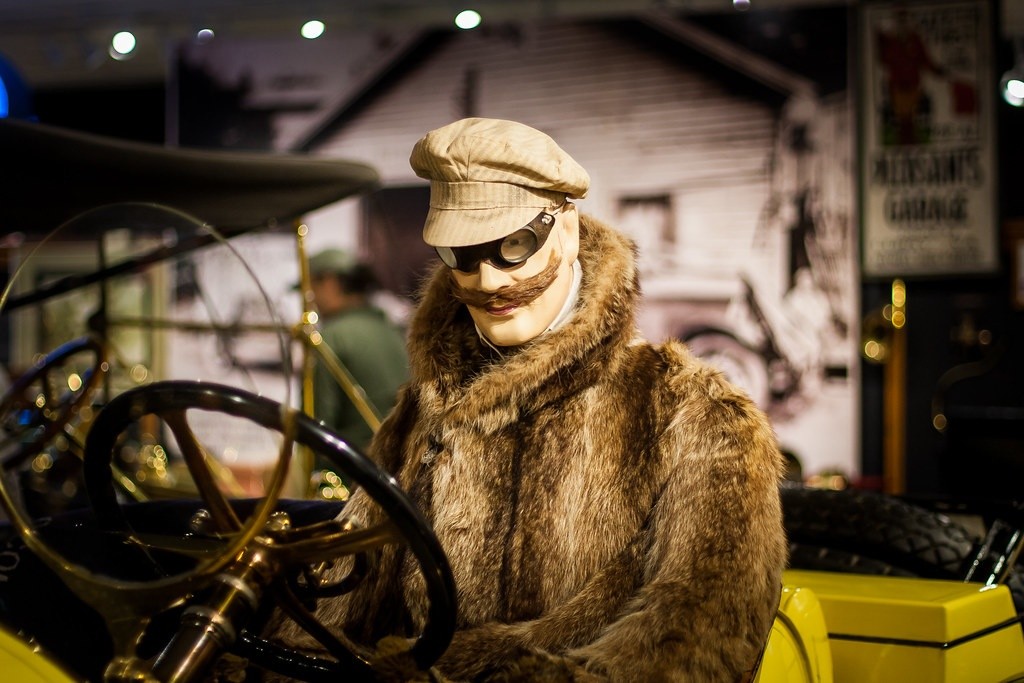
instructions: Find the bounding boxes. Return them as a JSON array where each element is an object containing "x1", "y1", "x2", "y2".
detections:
[{"x1": 431, "y1": 212, "x2": 567, "y2": 274}]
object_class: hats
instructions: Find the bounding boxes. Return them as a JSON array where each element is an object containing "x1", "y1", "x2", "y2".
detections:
[{"x1": 411, "y1": 117, "x2": 594, "y2": 248}]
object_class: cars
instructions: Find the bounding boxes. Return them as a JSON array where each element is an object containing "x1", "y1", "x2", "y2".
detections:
[{"x1": 0, "y1": 369, "x2": 1024, "y2": 683}]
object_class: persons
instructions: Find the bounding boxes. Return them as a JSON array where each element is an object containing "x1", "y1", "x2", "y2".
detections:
[
  {"x1": 286, "y1": 246, "x2": 416, "y2": 496},
  {"x1": 252, "y1": 114, "x2": 788, "y2": 682}
]
